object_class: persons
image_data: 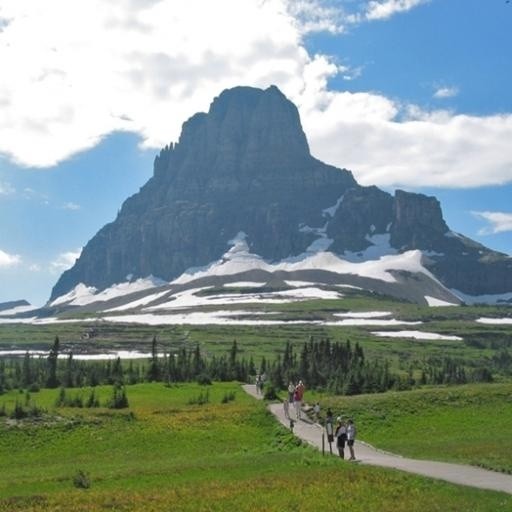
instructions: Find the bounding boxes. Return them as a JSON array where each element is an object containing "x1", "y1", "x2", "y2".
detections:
[{"x1": 282, "y1": 379, "x2": 356, "y2": 463}]
